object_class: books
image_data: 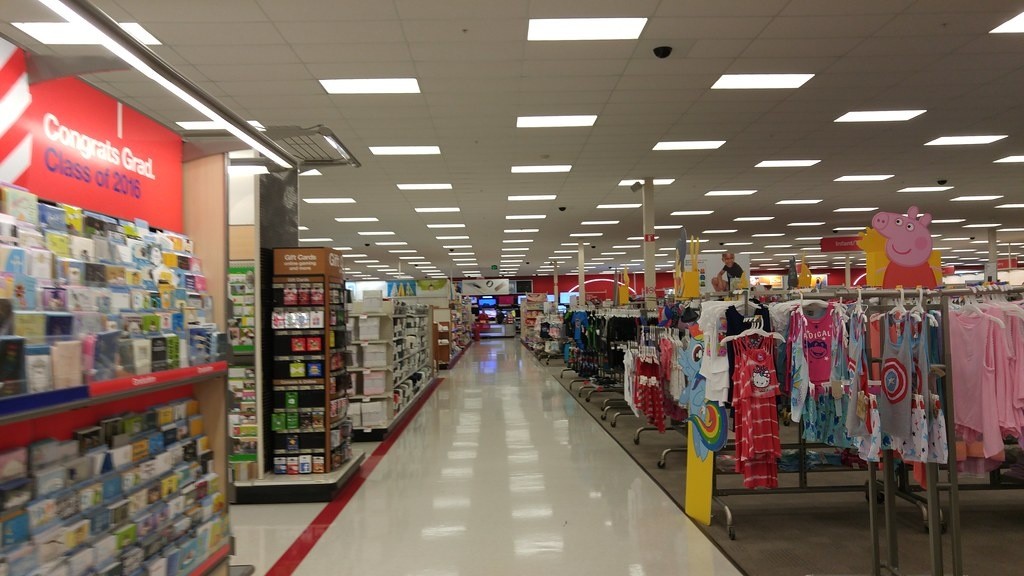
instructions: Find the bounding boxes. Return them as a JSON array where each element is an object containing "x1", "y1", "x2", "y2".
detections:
[
  {"x1": 0, "y1": 397, "x2": 230, "y2": 576},
  {"x1": 0, "y1": 181, "x2": 229, "y2": 397}
]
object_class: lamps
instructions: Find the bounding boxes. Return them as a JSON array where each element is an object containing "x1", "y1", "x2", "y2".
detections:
[{"x1": 39, "y1": 0, "x2": 300, "y2": 170}]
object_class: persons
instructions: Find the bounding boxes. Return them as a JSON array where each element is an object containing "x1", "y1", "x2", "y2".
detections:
[{"x1": 711, "y1": 254, "x2": 744, "y2": 301}]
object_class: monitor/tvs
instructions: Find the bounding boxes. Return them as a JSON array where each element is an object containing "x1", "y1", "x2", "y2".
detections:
[
  {"x1": 557, "y1": 304, "x2": 567, "y2": 314},
  {"x1": 560, "y1": 291, "x2": 581, "y2": 305},
  {"x1": 478, "y1": 309, "x2": 498, "y2": 318},
  {"x1": 471, "y1": 307, "x2": 478, "y2": 315},
  {"x1": 517, "y1": 295, "x2": 527, "y2": 305},
  {"x1": 546, "y1": 294, "x2": 556, "y2": 303},
  {"x1": 653, "y1": 46, "x2": 672, "y2": 58},
  {"x1": 477, "y1": 298, "x2": 498, "y2": 308}
]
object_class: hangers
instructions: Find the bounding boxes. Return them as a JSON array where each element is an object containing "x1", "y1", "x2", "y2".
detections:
[{"x1": 571, "y1": 284, "x2": 1024, "y2": 365}]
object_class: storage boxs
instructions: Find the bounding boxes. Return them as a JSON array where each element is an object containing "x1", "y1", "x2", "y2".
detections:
[{"x1": 271, "y1": 287, "x2": 352, "y2": 474}]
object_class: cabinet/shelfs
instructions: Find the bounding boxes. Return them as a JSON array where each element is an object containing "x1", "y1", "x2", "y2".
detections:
[
  {"x1": 432, "y1": 304, "x2": 472, "y2": 369},
  {"x1": 520, "y1": 302, "x2": 544, "y2": 352},
  {"x1": 349, "y1": 301, "x2": 439, "y2": 442},
  {"x1": 272, "y1": 247, "x2": 350, "y2": 474}
]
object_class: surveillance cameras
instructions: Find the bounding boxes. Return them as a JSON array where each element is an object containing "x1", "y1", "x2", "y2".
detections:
[
  {"x1": 346, "y1": 278, "x2": 349, "y2": 280},
  {"x1": 970, "y1": 237, "x2": 975, "y2": 239},
  {"x1": 365, "y1": 244, "x2": 370, "y2": 246},
  {"x1": 720, "y1": 243, "x2": 723, "y2": 245},
  {"x1": 526, "y1": 262, "x2": 529, "y2": 264},
  {"x1": 630, "y1": 182, "x2": 644, "y2": 193},
  {"x1": 415, "y1": 265, "x2": 418, "y2": 266},
  {"x1": 559, "y1": 206, "x2": 566, "y2": 211},
  {"x1": 591, "y1": 246, "x2": 596, "y2": 248},
  {"x1": 609, "y1": 267, "x2": 611, "y2": 269},
  {"x1": 450, "y1": 248, "x2": 454, "y2": 251},
  {"x1": 833, "y1": 230, "x2": 838, "y2": 233},
  {"x1": 425, "y1": 277, "x2": 428, "y2": 278},
  {"x1": 937, "y1": 180, "x2": 947, "y2": 185},
  {"x1": 550, "y1": 262, "x2": 556, "y2": 267}
]
